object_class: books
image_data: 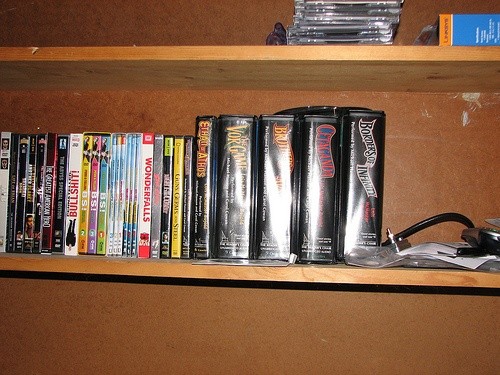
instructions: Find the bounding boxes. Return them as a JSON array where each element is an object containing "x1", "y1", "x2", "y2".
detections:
[
  {"x1": 257, "y1": 114, "x2": 300, "y2": 261},
  {"x1": 397, "y1": 241, "x2": 474, "y2": 256},
  {"x1": 302, "y1": 115, "x2": 339, "y2": 264},
  {"x1": 217, "y1": 114, "x2": 258, "y2": 260},
  {"x1": 0, "y1": 132, "x2": 194, "y2": 260},
  {"x1": 192, "y1": 115, "x2": 216, "y2": 260},
  {"x1": 340, "y1": 110, "x2": 385, "y2": 260}
]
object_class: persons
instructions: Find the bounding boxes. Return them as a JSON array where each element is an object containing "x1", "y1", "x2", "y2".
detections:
[
  {"x1": 83, "y1": 136, "x2": 90, "y2": 159},
  {"x1": 66, "y1": 219, "x2": 76, "y2": 251},
  {"x1": 163, "y1": 233, "x2": 168, "y2": 242},
  {"x1": 25, "y1": 215, "x2": 34, "y2": 237},
  {"x1": 92, "y1": 137, "x2": 99, "y2": 158},
  {"x1": 101, "y1": 138, "x2": 107, "y2": 158},
  {"x1": 1, "y1": 158, "x2": 8, "y2": 169},
  {"x1": 2, "y1": 139, "x2": 9, "y2": 150}
]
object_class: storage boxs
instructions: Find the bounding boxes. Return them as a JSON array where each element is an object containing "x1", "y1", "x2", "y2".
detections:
[{"x1": 431, "y1": 14, "x2": 500, "y2": 46}]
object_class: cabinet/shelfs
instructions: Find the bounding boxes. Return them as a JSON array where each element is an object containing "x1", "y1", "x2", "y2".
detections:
[{"x1": 0, "y1": 46, "x2": 500, "y2": 288}]
